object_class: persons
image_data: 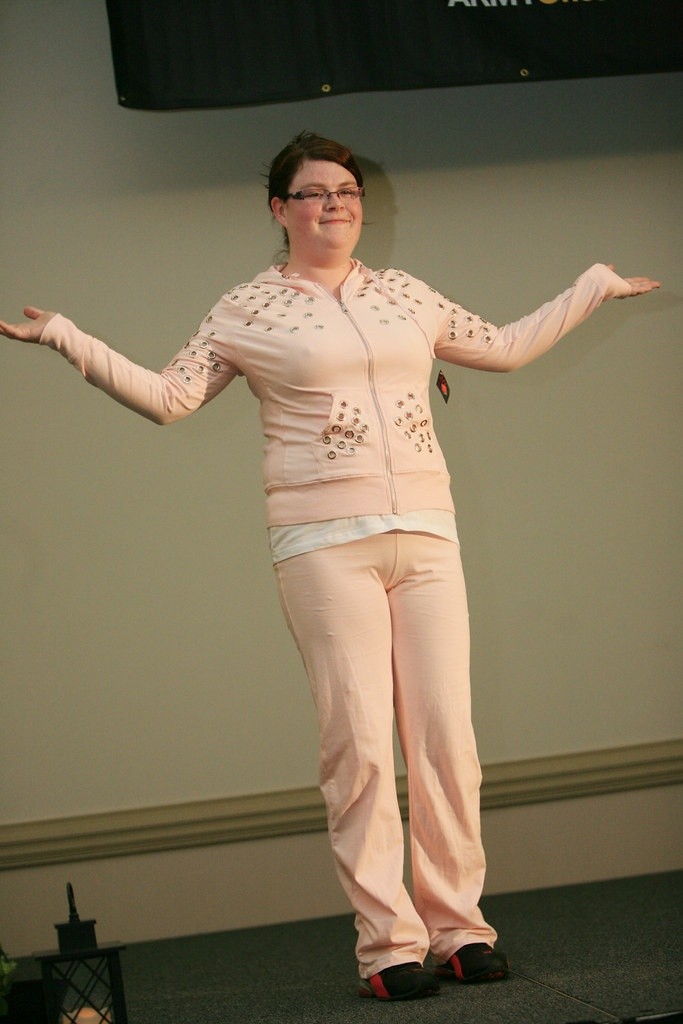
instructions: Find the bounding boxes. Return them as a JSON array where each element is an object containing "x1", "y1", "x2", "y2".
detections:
[{"x1": 1, "y1": 133, "x2": 663, "y2": 1001}]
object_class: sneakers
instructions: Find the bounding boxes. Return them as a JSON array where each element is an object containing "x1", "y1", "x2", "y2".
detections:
[
  {"x1": 358, "y1": 962, "x2": 440, "y2": 1001},
  {"x1": 434, "y1": 942, "x2": 508, "y2": 982}
]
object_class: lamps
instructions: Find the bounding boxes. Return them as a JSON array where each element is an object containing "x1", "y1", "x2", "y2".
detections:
[{"x1": 31, "y1": 879, "x2": 129, "y2": 1024}]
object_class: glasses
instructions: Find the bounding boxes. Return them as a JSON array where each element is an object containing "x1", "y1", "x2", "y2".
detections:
[{"x1": 277, "y1": 185, "x2": 365, "y2": 202}]
